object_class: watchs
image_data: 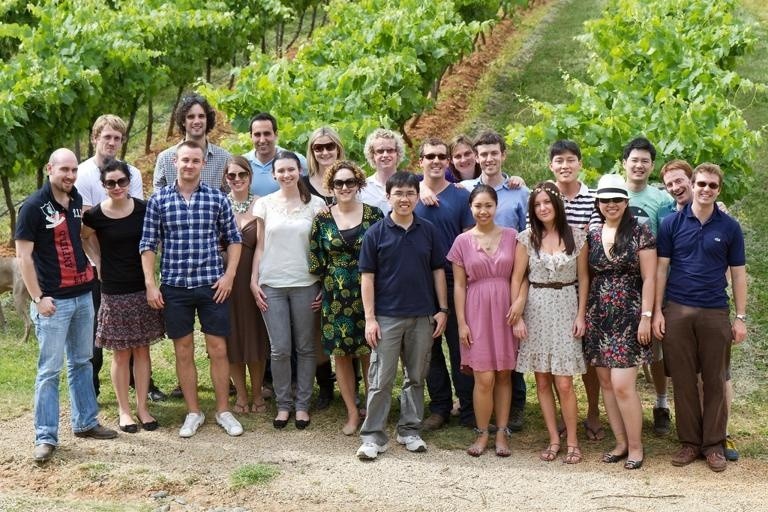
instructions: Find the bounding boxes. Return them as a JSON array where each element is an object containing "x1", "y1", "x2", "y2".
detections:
[
  {"x1": 641, "y1": 311, "x2": 653, "y2": 318},
  {"x1": 438, "y1": 308, "x2": 451, "y2": 314},
  {"x1": 735, "y1": 314, "x2": 747, "y2": 322},
  {"x1": 31, "y1": 293, "x2": 45, "y2": 303}
]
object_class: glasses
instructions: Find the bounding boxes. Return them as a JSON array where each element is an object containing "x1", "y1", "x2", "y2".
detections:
[
  {"x1": 388, "y1": 190, "x2": 418, "y2": 198},
  {"x1": 375, "y1": 148, "x2": 395, "y2": 155},
  {"x1": 332, "y1": 178, "x2": 357, "y2": 188},
  {"x1": 313, "y1": 141, "x2": 336, "y2": 152},
  {"x1": 697, "y1": 181, "x2": 719, "y2": 190},
  {"x1": 226, "y1": 170, "x2": 249, "y2": 181},
  {"x1": 423, "y1": 153, "x2": 448, "y2": 160},
  {"x1": 600, "y1": 197, "x2": 623, "y2": 204},
  {"x1": 103, "y1": 177, "x2": 131, "y2": 188}
]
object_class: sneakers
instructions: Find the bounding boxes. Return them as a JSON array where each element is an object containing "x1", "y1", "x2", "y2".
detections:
[
  {"x1": 726, "y1": 433, "x2": 739, "y2": 461},
  {"x1": 356, "y1": 439, "x2": 389, "y2": 460},
  {"x1": 180, "y1": 412, "x2": 205, "y2": 436},
  {"x1": 397, "y1": 430, "x2": 428, "y2": 452},
  {"x1": 213, "y1": 410, "x2": 244, "y2": 436},
  {"x1": 509, "y1": 406, "x2": 526, "y2": 431},
  {"x1": 652, "y1": 405, "x2": 674, "y2": 435}
]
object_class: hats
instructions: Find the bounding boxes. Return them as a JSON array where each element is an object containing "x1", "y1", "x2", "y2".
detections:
[{"x1": 586, "y1": 173, "x2": 632, "y2": 200}]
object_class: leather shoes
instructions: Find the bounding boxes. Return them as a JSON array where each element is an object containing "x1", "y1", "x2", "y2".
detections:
[
  {"x1": 149, "y1": 388, "x2": 167, "y2": 401},
  {"x1": 119, "y1": 415, "x2": 137, "y2": 432},
  {"x1": 706, "y1": 449, "x2": 729, "y2": 472},
  {"x1": 424, "y1": 413, "x2": 443, "y2": 430},
  {"x1": 31, "y1": 442, "x2": 57, "y2": 461},
  {"x1": 73, "y1": 424, "x2": 117, "y2": 439},
  {"x1": 172, "y1": 387, "x2": 181, "y2": 397},
  {"x1": 672, "y1": 444, "x2": 699, "y2": 467},
  {"x1": 136, "y1": 413, "x2": 159, "y2": 431},
  {"x1": 312, "y1": 395, "x2": 331, "y2": 411},
  {"x1": 273, "y1": 411, "x2": 290, "y2": 429},
  {"x1": 293, "y1": 411, "x2": 310, "y2": 430}
]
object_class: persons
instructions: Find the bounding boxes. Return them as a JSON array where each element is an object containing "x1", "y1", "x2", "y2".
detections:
[
  {"x1": 138, "y1": 141, "x2": 243, "y2": 439},
  {"x1": 248, "y1": 151, "x2": 322, "y2": 431},
  {"x1": 357, "y1": 129, "x2": 406, "y2": 422},
  {"x1": 357, "y1": 172, "x2": 450, "y2": 461},
  {"x1": 240, "y1": 113, "x2": 308, "y2": 402},
  {"x1": 414, "y1": 139, "x2": 476, "y2": 436},
  {"x1": 308, "y1": 160, "x2": 386, "y2": 436},
  {"x1": 446, "y1": 185, "x2": 529, "y2": 457},
  {"x1": 512, "y1": 181, "x2": 589, "y2": 464},
  {"x1": 657, "y1": 163, "x2": 748, "y2": 471},
  {"x1": 153, "y1": 93, "x2": 236, "y2": 397},
  {"x1": 584, "y1": 174, "x2": 656, "y2": 470},
  {"x1": 221, "y1": 156, "x2": 267, "y2": 415},
  {"x1": 660, "y1": 159, "x2": 740, "y2": 462},
  {"x1": 456, "y1": 132, "x2": 530, "y2": 429},
  {"x1": 622, "y1": 139, "x2": 677, "y2": 438},
  {"x1": 15, "y1": 147, "x2": 118, "y2": 463},
  {"x1": 80, "y1": 156, "x2": 165, "y2": 434},
  {"x1": 75, "y1": 112, "x2": 167, "y2": 402},
  {"x1": 544, "y1": 141, "x2": 605, "y2": 443},
  {"x1": 300, "y1": 125, "x2": 345, "y2": 410}
]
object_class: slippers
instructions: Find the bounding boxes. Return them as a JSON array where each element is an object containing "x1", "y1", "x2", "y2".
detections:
[
  {"x1": 558, "y1": 427, "x2": 568, "y2": 437},
  {"x1": 234, "y1": 402, "x2": 251, "y2": 413},
  {"x1": 253, "y1": 399, "x2": 267, "y2": 413},
  {"x1": 584, "y1": 424, "x2": 605, "y2": 441}
]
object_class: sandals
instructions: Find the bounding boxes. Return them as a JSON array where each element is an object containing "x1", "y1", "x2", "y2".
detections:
[
  {"x1": 624, "y1": 449, "x2": 647, "y2": 470},
  {"x1": 540, "y1": 443, "x2": 560, "y2": 462},
  {"x1": 495, "y1": 428, "x2": 512, "y2": 457},
  {"x1": 566, "y1": 445, "x2": 580, "y2": 464},
  {"x1": 600, "y1": 447, "x2": 628, "y2": 462},
  {"x1": 468, "y1": 431, "x2": 489, "y2": 456}
]
